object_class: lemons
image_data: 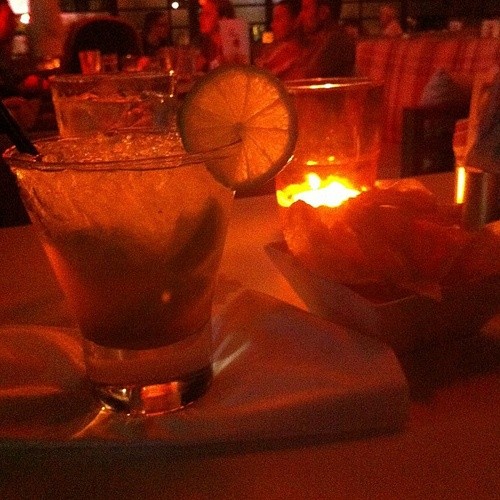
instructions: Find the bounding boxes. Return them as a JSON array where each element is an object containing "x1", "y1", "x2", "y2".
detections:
[
  {"x1": 50, "y1": 198, "x2": 224, "y2": 343},
  {"x1": 176, "y1": 63, "x2": 299, "y2": 190}
]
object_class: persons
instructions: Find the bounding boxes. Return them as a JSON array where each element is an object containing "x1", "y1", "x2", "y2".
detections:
[{"x1": 1, "y1": 1, "x2": 500, "y2": 86}]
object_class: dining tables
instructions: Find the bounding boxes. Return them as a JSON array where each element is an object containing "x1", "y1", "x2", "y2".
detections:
[{"x1": 0, "y1": 170, "x2": 500, "y2": 500}]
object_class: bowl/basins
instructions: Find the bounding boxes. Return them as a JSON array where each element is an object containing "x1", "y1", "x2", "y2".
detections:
[{"x1": 263, "y1": 225, "x2": 500, "y2": 356}]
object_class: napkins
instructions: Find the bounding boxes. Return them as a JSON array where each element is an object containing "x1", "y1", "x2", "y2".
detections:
[{"x1": 0, "y1": 290, "x2": 409, "y2": 456}]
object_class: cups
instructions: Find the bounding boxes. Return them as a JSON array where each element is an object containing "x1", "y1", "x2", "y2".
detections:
[
  {"x1": 78, "y1": 45, "x2": 197, "y2": 75},
  {"x1": 2, "y1": 128, "x2": 244, "y2": 418},
  {"x1": 273, "y1": 74, "x2": 395, "y2": 223},
  {"x1": 48, "y1": 70, "x2": 177, "y2": 139},
  {"x1": 481, "y1": 19, "x2": 500, "y2": 39}
]
